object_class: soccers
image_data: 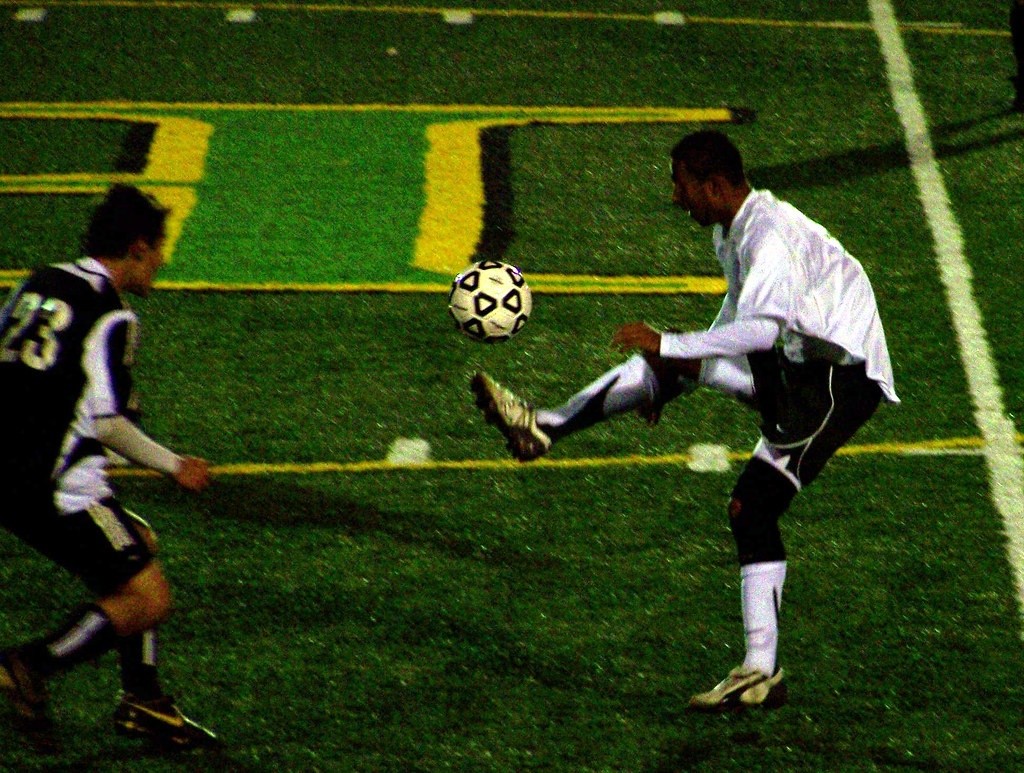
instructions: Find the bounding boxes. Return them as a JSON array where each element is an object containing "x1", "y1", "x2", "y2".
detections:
[{"x1": 448, "y1": 259, "x2": 536, "y2": 344}]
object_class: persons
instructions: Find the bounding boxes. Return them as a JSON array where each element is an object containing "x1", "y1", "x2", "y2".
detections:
[
  {"x1": 0, "y1": 179, "x2": 232, "y2": 754},
  {"x1": 469, "y1": 128, "x2": 905, "y2": 717}
]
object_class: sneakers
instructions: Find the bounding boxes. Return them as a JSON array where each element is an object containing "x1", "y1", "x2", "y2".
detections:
[
  {"x1": 687, "y1": 666, "x2": 786, "y2": 708},
  {"x1": 472, "y1": 371, "x2": 552, "y2": 463},
  {"x1": 119, "y1": 699, "x2": 219, "y2": 748},
  {"x1": 0, "y1": 647, "x2": 64, "y2": 754}
]
